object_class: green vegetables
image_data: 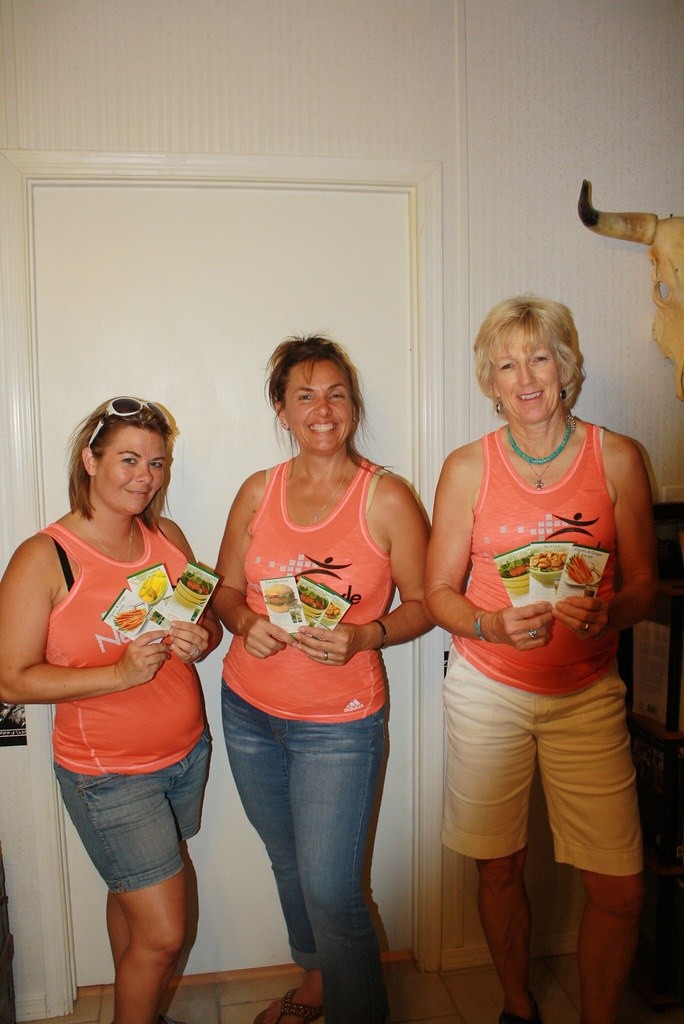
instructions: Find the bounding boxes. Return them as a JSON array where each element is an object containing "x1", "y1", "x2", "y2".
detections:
[
  {"x1": 297, "y1": 585, "x2": 329, "y2": 610},
  {"x1": 180, "y1": 571, "x2": 214, "y2": 596},
  {"x1": 499, "y1": 556, "x2": 530, "y2": 578}
]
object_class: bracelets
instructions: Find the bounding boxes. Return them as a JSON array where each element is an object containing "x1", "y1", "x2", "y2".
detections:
[
  {"x1": 372, "y1": 620, "x2": 386, "y2": 649},
  {"x1": 474, "y1": 613, "x2": 486, "y2": 642}
]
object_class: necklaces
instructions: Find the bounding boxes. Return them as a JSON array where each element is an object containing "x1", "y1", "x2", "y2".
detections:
[
  {"x1": 529, "y1": 462, "x2": 551, "y2": 489},
  {"x1": 296, "y1": 456, "x2": 347, "y2": 524},
  {"x1": 506, "y1": 415, "x2": 576, "y2": 463},
  {"x1": 103, "y1": 524, "x2": 134, "y2": 563}
]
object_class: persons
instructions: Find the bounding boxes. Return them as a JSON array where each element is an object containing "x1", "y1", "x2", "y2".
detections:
[
  {"x1": 423, "y1": 294, "x2": 659, "y2": 1023},
  {"x1": 0, "y1": 395, "x2": 224, "y2": 1024},
  {"x1": 212, "y1": 336, "x2": 438, "y2": 1023}
]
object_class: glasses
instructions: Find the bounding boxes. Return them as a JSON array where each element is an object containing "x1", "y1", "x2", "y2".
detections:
[{"x1": 89, "y1": 398, "x2": 170, "y2": 445}]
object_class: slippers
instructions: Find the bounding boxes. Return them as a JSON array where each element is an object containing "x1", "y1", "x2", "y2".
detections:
[{"x1": 253, "y1": 988, "x2": 323, "y2": 1024}]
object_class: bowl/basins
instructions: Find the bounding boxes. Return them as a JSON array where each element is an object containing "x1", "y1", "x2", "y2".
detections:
[
  {"x1": 299, "y1": 600, "x2": 325, "y2": 624},
  {"x1": 173, "y1": 580, "x2": 209, "y2": 609},
  {"x1": 321, "y1": 617, "x2": 339, "y2": 626},
  {"x1": 501, "y1": 573, "x2": 531, "y2": 596},
  {"x1": 531, "y1": 571, "x2": 563, "y2": 588},
  {"x1": 149, "y1": 583, "x2": 168, "y2": 606}
]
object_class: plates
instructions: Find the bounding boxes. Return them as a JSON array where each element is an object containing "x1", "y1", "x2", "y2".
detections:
[
  {"x1": 129, "y1": 602, "x2": 148, "y2": 635},
  {"x1": 564, "y1": 570, "x2": 601, "y2": 586}
]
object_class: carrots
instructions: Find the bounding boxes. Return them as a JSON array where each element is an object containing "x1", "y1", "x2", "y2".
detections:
[
  {"x1": 567, "y1": 556, "x2": 594, "y2": 584},
  {"x1": 115, "y1": 608, "x2": 147, "y2": 631}
]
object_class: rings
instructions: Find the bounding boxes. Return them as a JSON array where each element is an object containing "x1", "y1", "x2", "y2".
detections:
[
  {"x1": 528, "y1": 630, "x2": 537, "y2": 639},
  {"x1": 586, "y1": 623, "x2": 589, "y2": 631},
  {"x1": 324, "y1": 653, "x2": 328, "y2": 659},
  {"x1": 191, "y1": 645, "x2": 199, "y2": 656}
]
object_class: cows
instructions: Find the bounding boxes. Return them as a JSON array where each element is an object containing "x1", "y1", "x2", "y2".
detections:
[{"x1": 576, "y1": 178, "x2": 684, "y2": 403}]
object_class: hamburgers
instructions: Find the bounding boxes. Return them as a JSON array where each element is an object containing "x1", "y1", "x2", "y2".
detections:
[{"x1": 264, "y1": 584, "x2": 299, "y2": 613}]
